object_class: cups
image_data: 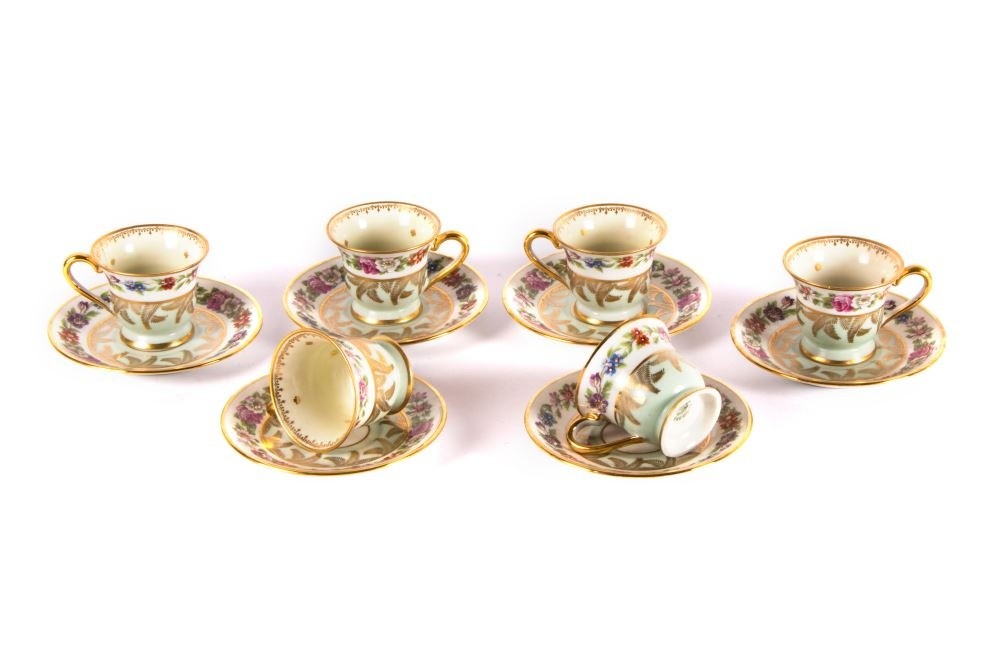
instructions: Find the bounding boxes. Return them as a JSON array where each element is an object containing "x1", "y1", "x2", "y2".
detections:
[
  {"x1": 62, "y1": 223, "x2": 209, "y2": 352},
  {"x1": 523, "y1": 203, "x2": 668, "y2": 325},
  {"x1": 564, "y1": 313, "x2": 723, "y2": 458},
  {"x1": 783, "y1": 235, "x2": 932, "y2": 367},
  {"x1": 326, "y1": 201, "x2": 471, "y2": 327},
  {"x1": 268, "y1": 328, "x2": 414, "y2": 456}
]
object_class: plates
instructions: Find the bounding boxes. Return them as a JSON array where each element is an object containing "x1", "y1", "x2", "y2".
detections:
[
  {"x1": 729, "y1": 285, "x2": 949, "y2": 387},
  {"x1": 522, "y1": 369, "x2": 753, "y2": 479},
  {"x1": 502, "y1": 250, "x2": 713, "y2": 346},
  {"x1": 219, "y1": 372, "x2": 448, "y2": 476},
  {"x1": 282, "y1": 253, "x2": 488, "y2": 346},
  {"x1": 46, "y1": 278, "x2": 264, "y2": 375}
]
